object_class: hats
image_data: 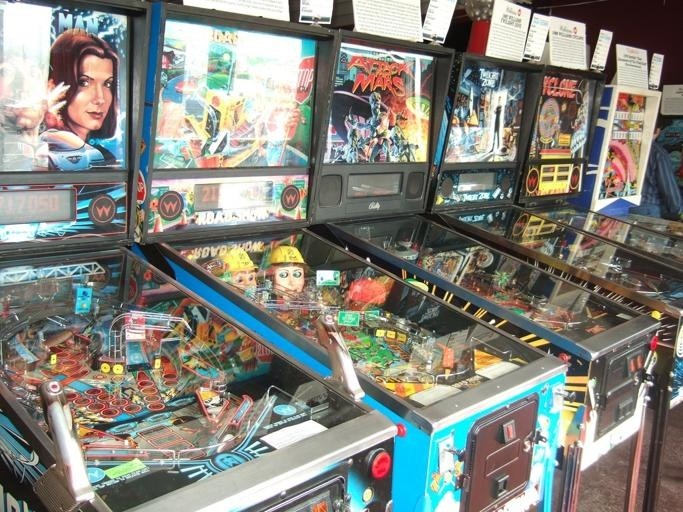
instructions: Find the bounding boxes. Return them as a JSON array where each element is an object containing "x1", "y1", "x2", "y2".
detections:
[
  {"x1": 215, "y1": 246, "x2": 259, "y2": 273},
  {"x1": 269, "y1": 246, "x2": 305, "y2": 264}
]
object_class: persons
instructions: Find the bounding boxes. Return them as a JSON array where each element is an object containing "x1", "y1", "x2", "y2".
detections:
[
  {"x1": 0, "y1": 27, "x2": 119, "y2": 172},
  {"x1": 628, "y1": 113, "x2": 682, "y2": 222},
  {"x1": 490, "y1": 96, "x2": 502, "y2": 153},
  {"x1": 365, "y1": 92, "x2": 388, "y2": 164}
]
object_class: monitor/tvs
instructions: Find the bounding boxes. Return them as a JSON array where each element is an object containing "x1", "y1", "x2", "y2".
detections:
[
  {"x1": 444, "y1": 62, "x2": 528, "y2": 164},
  {"x1": 324, "y1": 42, "x2": 435, "y2": 163},
  {"x1": 0, "y1": 0, "x2": 131, "y2": 174},
  {"x1": 153, "y1": 20, "x2": 317, "y2": 169},
  {"x1": 531, "y1": 73, "x2": 585, "y2": 158},
  {"x1": 597, "y1": 93, "x2": 647, "y2": 199}
]
